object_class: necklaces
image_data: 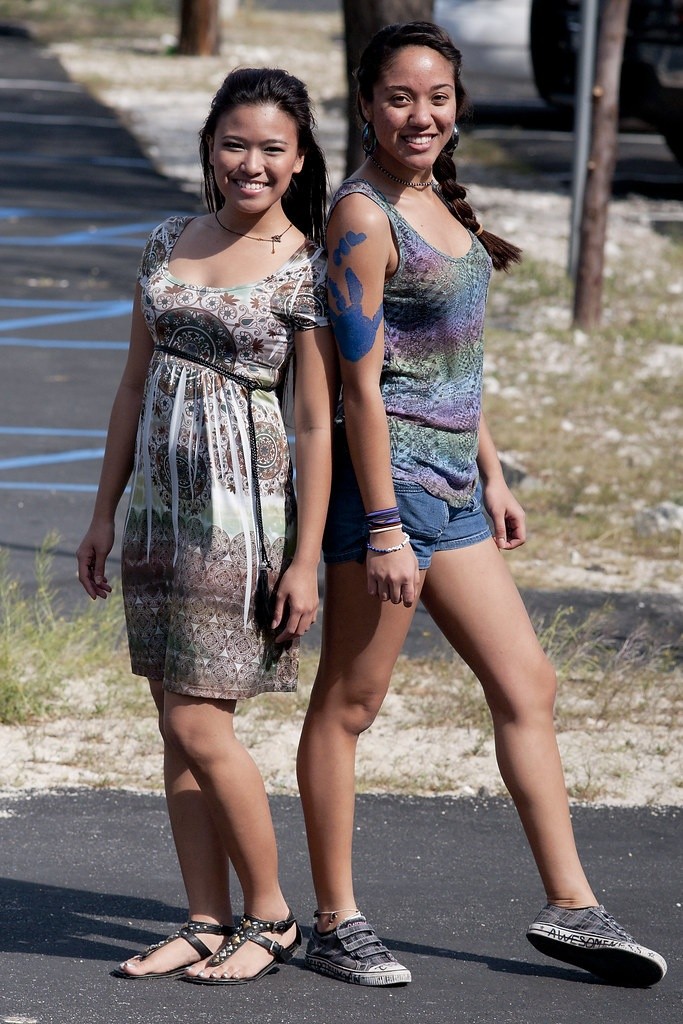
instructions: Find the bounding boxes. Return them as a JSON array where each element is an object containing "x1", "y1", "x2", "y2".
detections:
[
  {"x1": 364, "y1": 151, "x2": 442, "y2": 195},
  {"x1": 215, "y1": 207, "x2": 293, "y2": 255}
]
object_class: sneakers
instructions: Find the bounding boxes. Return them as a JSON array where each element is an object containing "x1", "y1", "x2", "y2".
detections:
[
  {"x1": 527, "y1": 901, "x2": 668, "y2": 987},
  {"x1": 305, "y1": 918, "x2": 410, "y2": 988}
]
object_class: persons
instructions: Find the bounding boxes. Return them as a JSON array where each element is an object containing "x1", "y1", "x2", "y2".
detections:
[
  {"x1": 297, "y1": 21, "x2": 667, "y2": 987},
  {"x1": 75, "y1": 66, "x2": 332, "y2": 986}
]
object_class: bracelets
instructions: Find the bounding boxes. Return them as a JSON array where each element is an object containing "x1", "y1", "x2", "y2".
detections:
[{"x1": 361, "y1": 507, "x2": 410, "y2": 554}]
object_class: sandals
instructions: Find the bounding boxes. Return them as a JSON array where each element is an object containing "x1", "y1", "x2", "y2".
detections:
[{"x1": 117, "y1": 914, "x2": 302, "y2": 985}]
